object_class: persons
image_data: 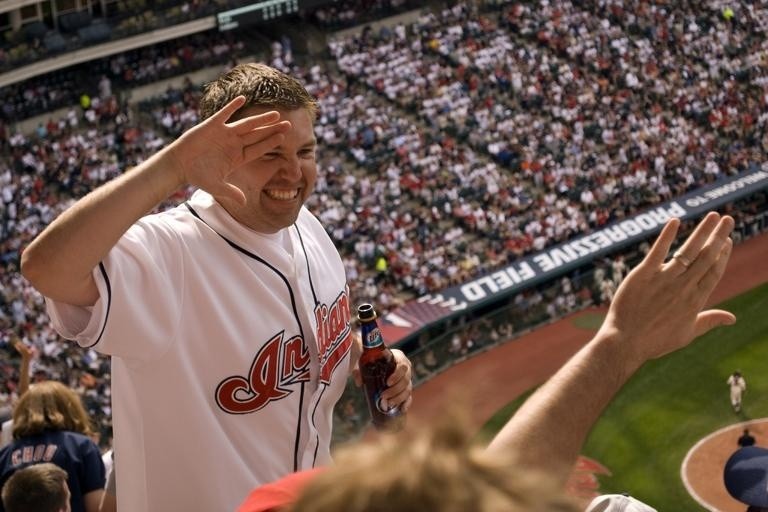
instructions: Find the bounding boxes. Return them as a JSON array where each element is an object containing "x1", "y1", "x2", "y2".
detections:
[{"x1": 0, "y1": 0, "x2": 767, "y2": 511}]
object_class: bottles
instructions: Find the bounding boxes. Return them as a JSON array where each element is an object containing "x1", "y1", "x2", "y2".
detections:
[{"x1": 354, "y1": 301, "x2": 406, "y2": 438}]
